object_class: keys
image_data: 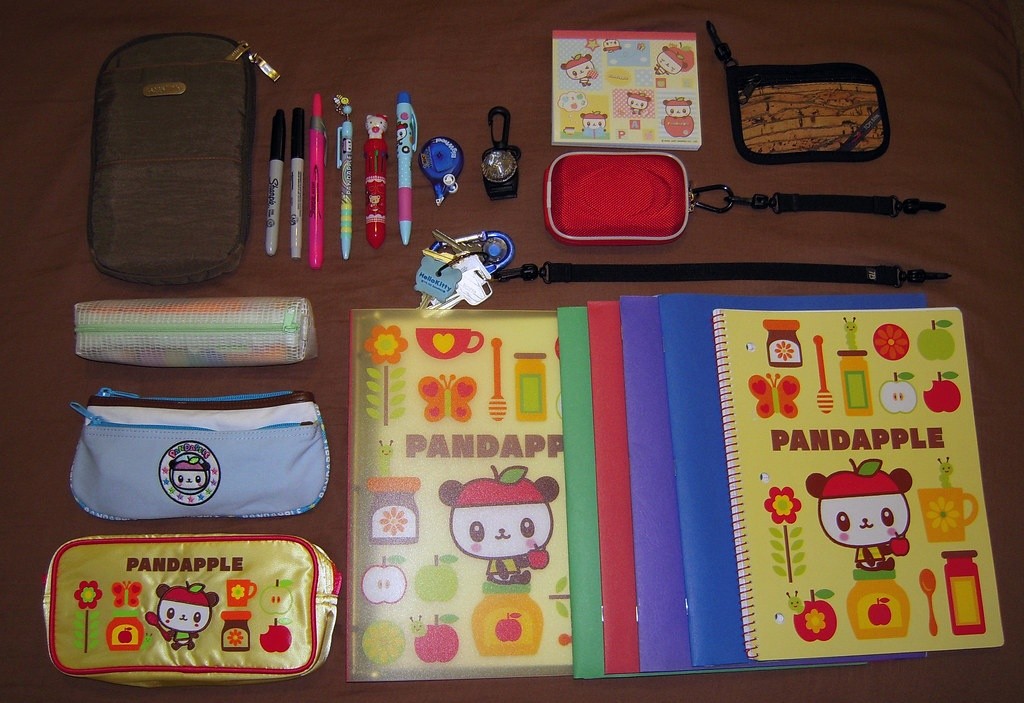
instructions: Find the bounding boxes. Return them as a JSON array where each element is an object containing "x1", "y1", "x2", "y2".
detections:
[{"x1": 414, "y1": 229, "x2": 516, "y2": 319}]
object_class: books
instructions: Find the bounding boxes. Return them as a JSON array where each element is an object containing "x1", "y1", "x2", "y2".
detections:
[
  {"x1": 551, "y1": 30, "x2": 702, "y2": 151},
  {"x1": 345, "y1": 291, "x2": 1006, "y2": 682}
]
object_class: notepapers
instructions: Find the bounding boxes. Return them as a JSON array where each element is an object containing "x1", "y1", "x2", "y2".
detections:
[{"x1": 551, "y1": 29, "x2": 702, "y2": 151}]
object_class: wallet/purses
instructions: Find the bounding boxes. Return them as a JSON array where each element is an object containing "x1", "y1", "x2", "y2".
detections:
[{"x1": 87, "y1": 33, "x2": 281, "y2": 287}]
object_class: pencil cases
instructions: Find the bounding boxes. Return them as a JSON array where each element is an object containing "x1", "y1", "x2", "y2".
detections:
[
  {"x1": 72, "y1": 296, "x2": 318, "y2": 367},
  {"x1": 69, "y1": 387, "x2": 330, "y2": 520},
  {"x1": 41, "y1": 533, "x2": 343, "y2": 687}
]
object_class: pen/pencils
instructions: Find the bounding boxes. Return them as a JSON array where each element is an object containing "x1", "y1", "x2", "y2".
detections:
[
  {"x1": 290, "y1": 107, "x2": 306, "y2": 259},
  {"x1": 365, "y1": 114, "x2": 388, "y2": 246},
  {"x1": 337, "y1": 106, "x2": 353, "y2": 260},
  {"x1": 310, "y1": 92, "x2": 328, "y2": 269},
  {"x1": 396, "y1": 92, "x2": 417, "y2": 246},
  {"x1": 265, "y1": 108, "x2": 286, "y2": 257}
]
